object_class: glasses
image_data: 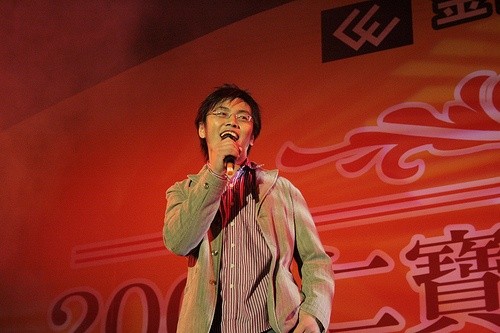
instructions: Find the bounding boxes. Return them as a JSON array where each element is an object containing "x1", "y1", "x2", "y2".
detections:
[{"x1": 207, "y1": 109, "x2": 257, "y2": 125}]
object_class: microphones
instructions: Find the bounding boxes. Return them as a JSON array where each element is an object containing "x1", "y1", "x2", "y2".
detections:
[{"x1": 222, "y1": 133, "x2": 236, "y2": 175}]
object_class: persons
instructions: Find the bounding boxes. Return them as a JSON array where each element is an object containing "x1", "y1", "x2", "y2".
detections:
[{"x1": 162, "y1": 83, "x2": 335, "y2": 333}]
